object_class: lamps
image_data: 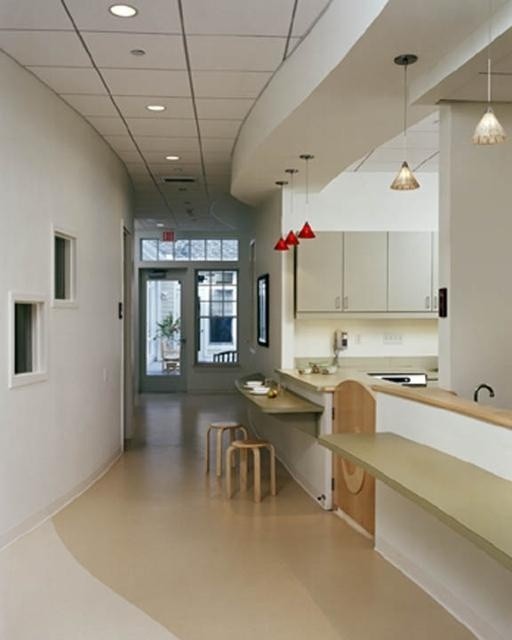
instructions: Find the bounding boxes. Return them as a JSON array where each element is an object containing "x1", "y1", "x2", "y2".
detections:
[
  {"x1": 473, "y1": 103, "x2": 509, "y2": 147},
  {"x1": 285, "y1": 230, "x2": 301, "y2": 246},
  {"x1": 273, "y1": 237, "x2": 289, "y2": 250},
  {"x1": 298, "y1": 221, "x2": 317, "y2": 239},
  {"x1": 391, "y1": 161, "x2": 421, "y2": 191}
]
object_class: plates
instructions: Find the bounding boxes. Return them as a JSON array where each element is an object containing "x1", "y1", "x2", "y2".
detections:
[
  {"x1": 243, "y1": 385, "x2": 265, "y2": 389},
  {"x1": 249, "y1": 390, "x2": 270, "y2": 394}
]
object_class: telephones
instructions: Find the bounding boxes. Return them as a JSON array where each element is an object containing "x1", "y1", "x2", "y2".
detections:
[{"x1": 334, "y1": 330, "x2": 349, "y2": 351}]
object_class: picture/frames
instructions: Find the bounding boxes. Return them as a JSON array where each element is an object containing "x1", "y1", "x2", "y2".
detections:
[{"x1": 256, "y1": 274, "x2": 269, "y2": 347}]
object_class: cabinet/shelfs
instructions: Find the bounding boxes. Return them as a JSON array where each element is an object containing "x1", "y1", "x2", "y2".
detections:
[
  {"x1": 296, "y1": 231, "x2": 387, "y2": 320},
  {"x1": 275, "y1": 355, "x2": 438, "y2": 513},
  {"x1": 388, "y1": 230, "x2": 439, "y2": 319}
]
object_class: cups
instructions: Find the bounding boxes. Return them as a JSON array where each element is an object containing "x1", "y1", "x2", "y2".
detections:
[
  {"x1": 265, "y1": 378, "x2": 273, "y2": 388},
  {"x1": 253, "y1": 387, "x2": 270, "y2": 391},
  {"x1": 277, "y1": 383, "x2": 286, "y2": 399},
  {"x1": 246, "y1": 381, "x2": 263, "y2": 386}
]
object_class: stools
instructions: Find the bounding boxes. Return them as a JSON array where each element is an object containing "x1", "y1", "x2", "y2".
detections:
[
  {"x1": 205, "y1": 423, "x2": 247, "y2": 475},
  {"x1": 226, "y1": 440, "x2": 277, "y2": 504}
]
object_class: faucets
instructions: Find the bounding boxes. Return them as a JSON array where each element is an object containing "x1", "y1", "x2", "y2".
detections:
[{"x1": 474, "y1": 383, "x2": 495, "y2": 403}]
